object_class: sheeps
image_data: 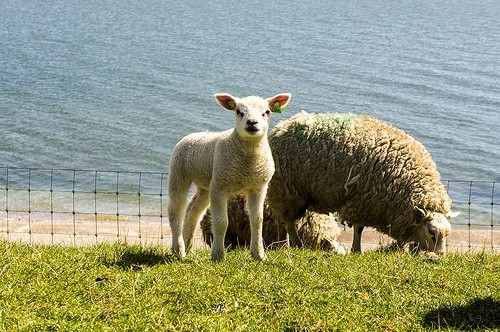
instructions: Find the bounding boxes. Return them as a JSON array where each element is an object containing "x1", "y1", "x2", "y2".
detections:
[
  {"x1": 265, "y1": 111, "x2": 461, "y2": 256},
  {"x1": 166, "y1": 91, "x2": 292, "y2": 263},
  {"x1": 199, "y1": 191, "x2": 346, "y2": 255}
]
